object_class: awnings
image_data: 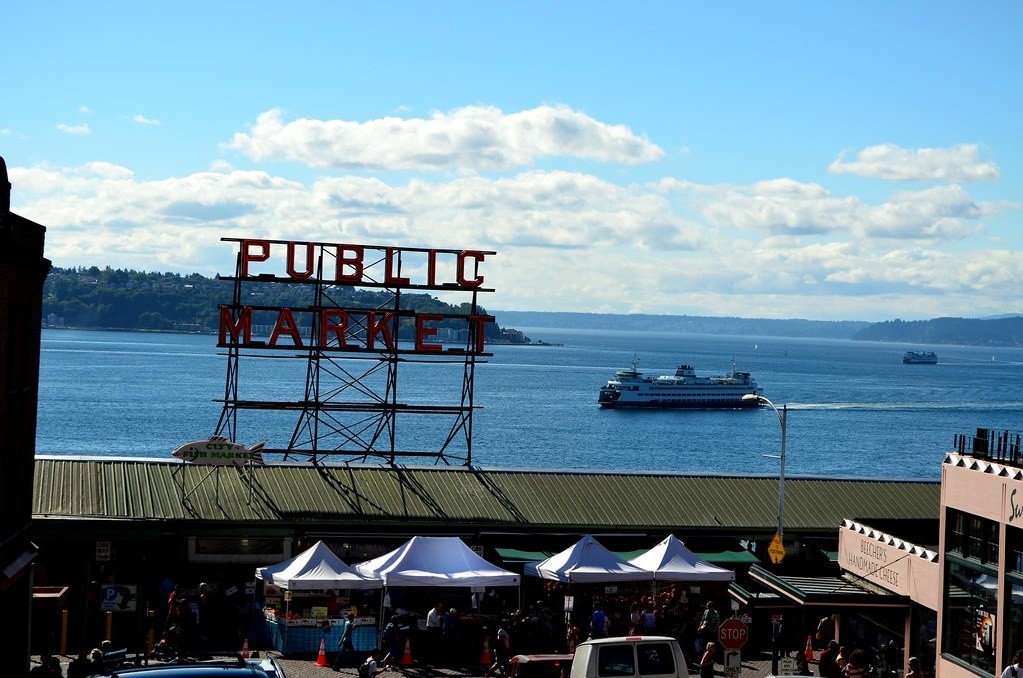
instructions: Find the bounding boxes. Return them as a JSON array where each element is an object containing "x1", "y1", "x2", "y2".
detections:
[
  {"x1": 494, "y1": 544, "x2": 761, "y2": 566},
  {"x1": 817, "y1": 548, "x2": 841, "y2": 561}
]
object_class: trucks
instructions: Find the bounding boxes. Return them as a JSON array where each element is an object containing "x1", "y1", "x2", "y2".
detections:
[{"x1": 570, "y1": 636, "x2": 691, "y2": 678}]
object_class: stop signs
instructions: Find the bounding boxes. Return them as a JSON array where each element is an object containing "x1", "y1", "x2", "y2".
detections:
[{"x1": 717, "y1": 618, "x2": 749, "y2": 650}]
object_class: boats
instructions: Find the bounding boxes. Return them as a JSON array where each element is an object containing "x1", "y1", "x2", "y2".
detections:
[
  {"x1": 903, "y1": 350, "x2": 938, "y2": 365},
  {"x1": 597, "y1": 350, "x2": 767, "y2": 410}
]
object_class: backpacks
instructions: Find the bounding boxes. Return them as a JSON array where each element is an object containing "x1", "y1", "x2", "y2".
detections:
[
  {"x1": 359, "y1": 659, "x2": 375, "y2": 678},
  {"x1": 382, "y1": 625, "x2": 397, "y2": 645}
]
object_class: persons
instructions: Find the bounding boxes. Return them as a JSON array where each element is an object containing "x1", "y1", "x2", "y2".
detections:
[{"x1": 30, "y1": 579, "x2": 1023, "y2": 678}]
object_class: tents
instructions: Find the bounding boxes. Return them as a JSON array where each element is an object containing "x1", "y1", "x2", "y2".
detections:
[
  {"x1": 525, "y1": 535, "x2": 654, "y2": 609},
  {"x1": 628, "y1": 535, "x2": 739, "y2": 619},
  {"x1": 352, "y1": 537, "x2": 522, "y2": 662},
  {"x1": 255, "y1": 541, "x2": 384, "y2": 655}
]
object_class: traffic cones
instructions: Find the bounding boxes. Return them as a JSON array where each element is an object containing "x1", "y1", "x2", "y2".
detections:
[
  {"x1": 805, "y1": 636, "x2": 815, "y2": 663},
  {"x1": 313, "y1": 638, "x2": 332, "y2": 667},
  {"x1": 479, "y1": 635, "x2": 494, "y2": 668},
  {"x1": 240, "y1": 638, "x2": 250, "y2": 658},
  {"x1": 397, "y1": 639, "x2": 416, "y2": 666}
]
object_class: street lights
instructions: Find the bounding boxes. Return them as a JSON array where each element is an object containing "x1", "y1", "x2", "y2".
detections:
[{"x1": 741, "y1": 392, "x2": 788, "y2": 678}]
